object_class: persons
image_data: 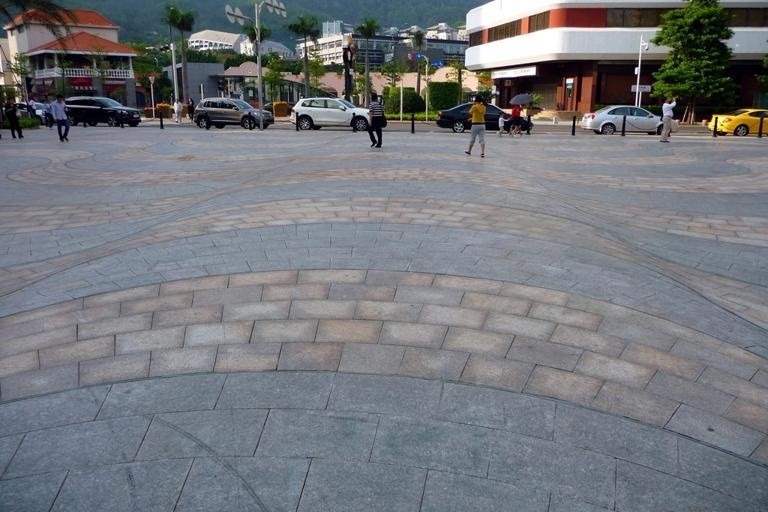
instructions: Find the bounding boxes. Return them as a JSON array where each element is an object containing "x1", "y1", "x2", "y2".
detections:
[
  {"x1": 497, "y1": 111, "x2": 510, "y2": 137},
  {"x1": 0, "y1": 94, "x2": 70, "y2": 141},
  {"x1": 366, "y1": 94, "x2": 384, "y2": 148},
  {"x1": 464, "y1": 95, "x2": 486, "y2": 157},
  {"x1": 511, "y1": 105, "x2": 524, "y2": 136},
  {"x1": 660, "y1": 97, "x2": 676, "y2": 142},
  {"x1": 174, "y1": 97, "x2": 183, "y2": 123},
  {"x1": 186, "y1": 97, "x2": 194, "y2": 123}
]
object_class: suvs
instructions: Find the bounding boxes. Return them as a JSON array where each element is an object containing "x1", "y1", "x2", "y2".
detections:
[
  {"x1": 64, "y1": 96, "x2": 141, "y2": 127},
  {"x1": 192, "y1": 97, "x2": 274, "y2": 129},
  {"x1": 289, "y1": 97, "x2": 386, "y2": 130}
]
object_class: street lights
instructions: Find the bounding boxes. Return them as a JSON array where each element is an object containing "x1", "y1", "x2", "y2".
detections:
[
  {"x1": 394, "y1": 76, "x2": 403, "y2": 121},
  {"x1": 633, "y1": 32, "x2": 648, "y2": 107}
]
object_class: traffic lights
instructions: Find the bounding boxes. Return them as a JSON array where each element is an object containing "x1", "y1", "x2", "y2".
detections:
[
  {"x1": 25, "y1": 77, "x2": 33, "y2": 93},
  {"x1": 216, "y1": 77, "x2": 224, "y2": 90},
  {"x1": 159, "y1": 45, "x2": 170, "y2": 51}
]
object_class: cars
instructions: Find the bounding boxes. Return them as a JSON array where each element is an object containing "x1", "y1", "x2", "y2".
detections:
[
  {"x1": 581, "y1": 105, "x2": 678, "y2": 136},
  {"x1": 435, "y1": 102, "x2": 533, "y2": 133},
  {"x1": 707, "y1": 108, "x2": 767, "y2": 136},
  {"x1": 14, "y1": 102, "x2": 46, "y2": 125}
]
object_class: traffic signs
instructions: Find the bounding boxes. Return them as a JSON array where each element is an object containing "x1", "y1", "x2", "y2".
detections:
[{"x1": 630, "y1": 83, "x2": 651, "y2": 93}]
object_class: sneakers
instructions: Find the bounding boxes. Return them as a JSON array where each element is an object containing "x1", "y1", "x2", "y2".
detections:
[
  {"x1": 480, "y1": 154, "x2": 485, "y2": 157},
  {"x1": 382, "y1": 116, "x2": 387, "y2": 128},
  {"x1": 371, "y1": 142, "x2": 376, "y2": 147},
  {"x1": 464, "y1": 151, "x2": 472, "y2": 155},
  {"x1": 378, "y1": 145, "x2": 382, "y2": 148}
]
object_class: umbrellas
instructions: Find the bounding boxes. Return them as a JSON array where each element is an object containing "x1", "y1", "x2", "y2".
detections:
[{"x1": 509, "y1": 93, "x2": 532, "y2": 111}]
